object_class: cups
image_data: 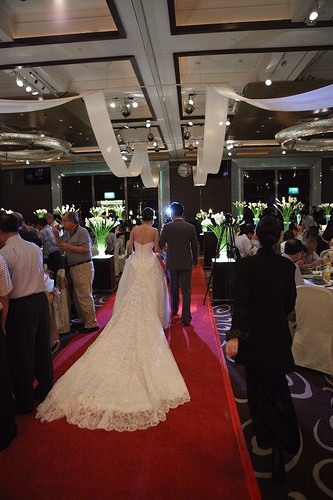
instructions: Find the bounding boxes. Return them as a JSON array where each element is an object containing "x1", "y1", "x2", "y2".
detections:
[{"x1": 316, "y1": 265, "x2": 322, "y2": 276}]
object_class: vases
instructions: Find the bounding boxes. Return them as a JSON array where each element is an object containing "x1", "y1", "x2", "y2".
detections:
[
  {"x1": 219, "y1": 237, "x2": 229, "y2": 262},
  {"x1": 283, "y1": 222, "x2": 292, "y2": 233},
  {"x1": 326, "y1": 215, "x2": 331, "y2": 225},
  {"x1": 93, "y1": 233, "x2": 108, "y2": 257},
  {"x1": 253, "y1": 218, "x2": 260, "y2": 232},
  {"x1": 296, "y1": 214, "x2": 301, "y2": 224}
]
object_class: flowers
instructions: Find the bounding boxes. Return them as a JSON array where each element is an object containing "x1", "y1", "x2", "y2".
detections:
[
  {"x1": 318, "y1": 202, "x2": 333, "y2": 215},
  {"x1": 84, "y1": 207, "x2": 124, "y2": 240},
  {"x1": 54, "y1": 203, "x2": 79, "y2": 217},
  {"x1": 33, "y1": 208, "x2": 48, "y2": 219},
  {"x1": 272, "y1": 196, "x2": 303, "y2": 222},
  {"x1": 195, "y1": 200, "x2": 268, "y2": 243}
]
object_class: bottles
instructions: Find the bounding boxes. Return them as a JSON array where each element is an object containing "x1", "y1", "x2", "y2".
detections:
[{"x1": 323, "y1": 266, "x2": 331, "y2": 281}]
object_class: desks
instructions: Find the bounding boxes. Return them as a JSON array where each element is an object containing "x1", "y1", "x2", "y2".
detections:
[
  {"x1": 303, "y1": 278, "x2": 333, "y2": 289},
  {"x1": 203, "y1": 231, "x2": 236, "y2": 301},
  {"x1": 93, "y1": 255, "x2": 117, "y2": 293}
]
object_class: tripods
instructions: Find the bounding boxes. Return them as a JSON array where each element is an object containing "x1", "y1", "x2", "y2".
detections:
[{"x1": 202, "y1": 223, "x2": 240, "y2": 305}]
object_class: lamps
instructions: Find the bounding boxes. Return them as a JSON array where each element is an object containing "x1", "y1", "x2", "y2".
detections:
[
  {"x1": 0, "y1": 132, "x2": 71, "y2": 162},
  {"x1": 275, "y1": 118, "x2": 333, "y2": 153}
]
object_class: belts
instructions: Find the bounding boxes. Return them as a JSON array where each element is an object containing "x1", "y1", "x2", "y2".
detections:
[{"x1": 70, "y1": 259, "x2": 91, "y2": 266}]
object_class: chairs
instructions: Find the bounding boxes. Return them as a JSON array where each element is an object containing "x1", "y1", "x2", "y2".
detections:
[
  {"x1": 113, "y1": 238, "x2": 130, "y2": 276},
  {"x1": 291, "y1": 284, "x2": 333, "y2": 376}
]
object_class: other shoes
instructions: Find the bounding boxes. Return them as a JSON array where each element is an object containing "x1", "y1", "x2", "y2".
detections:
[
  {"x1": 77, "y1": 326, "x2": 100, "y2": 333},
  {"x1": 72, "y1": 323, "x2": 84, "y2": 328},
  {"x1": 13, "y1": 389, "x2": 45, "y2": 413}
]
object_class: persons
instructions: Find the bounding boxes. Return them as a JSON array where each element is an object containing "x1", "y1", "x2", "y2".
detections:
[
  {"x1": 36, "y1": 208, "x2": 190, "y2": 431},
  {"x1": 223, "y1": 198, "x2": 333, "y2": 278},
  {"x1": 157, "y1": 202, "x2": 198, "y2": 325},
  {"x1": 141, "y1": 202, "x2": 145, "y2": 211},
  {"x1": 225, "y1": 216, "x2": 297, "y2": 449},
  {"x1": 86, "y1": 209, "x2": 143, "y2": 255},
  {"x1": 0, "y1": 210, "x2": 99, "y2": 450}
]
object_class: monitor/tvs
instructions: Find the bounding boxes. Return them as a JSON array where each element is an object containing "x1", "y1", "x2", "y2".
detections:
[{"x1": 24, "y1": 167, "x2": 51, "y2": 186}]
